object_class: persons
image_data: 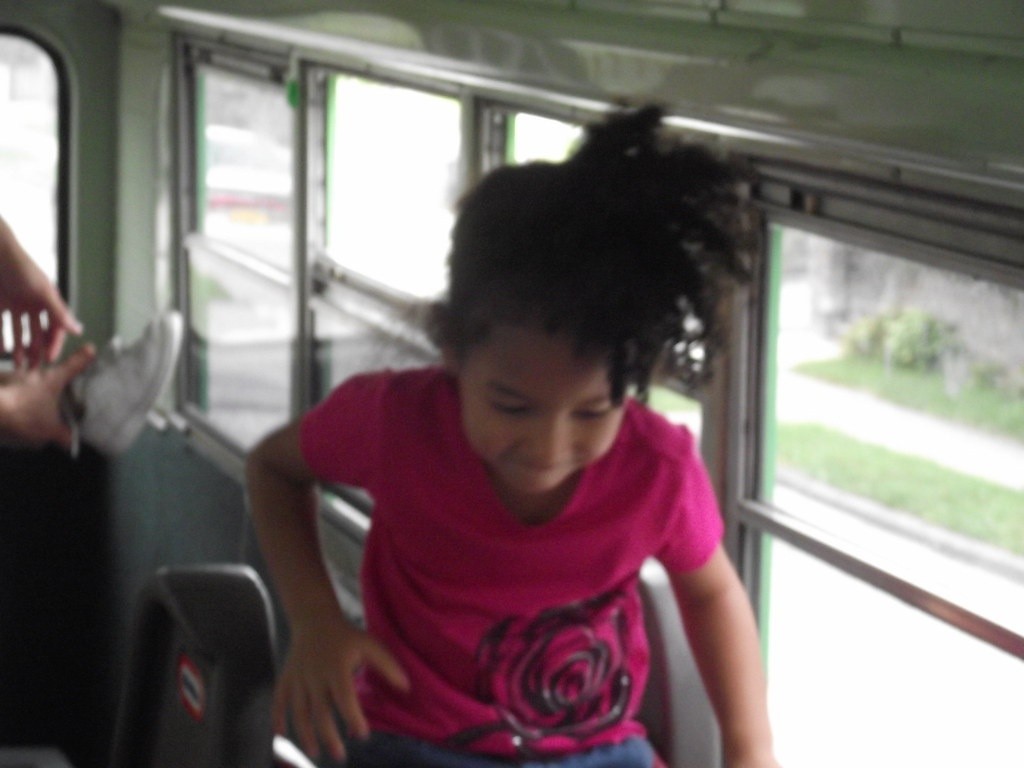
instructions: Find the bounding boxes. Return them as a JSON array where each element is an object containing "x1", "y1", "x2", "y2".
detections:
[
  {"x1": 0, "y1": 218, "x2": 186, "y2": 463},
  {"x1": 246, "y1": 101, "x2": 776, "y2": 766}
]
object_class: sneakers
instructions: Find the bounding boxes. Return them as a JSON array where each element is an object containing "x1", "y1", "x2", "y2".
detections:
[{"x1": 77, "y1": 308, "x2": 185, "y2": 451}]
